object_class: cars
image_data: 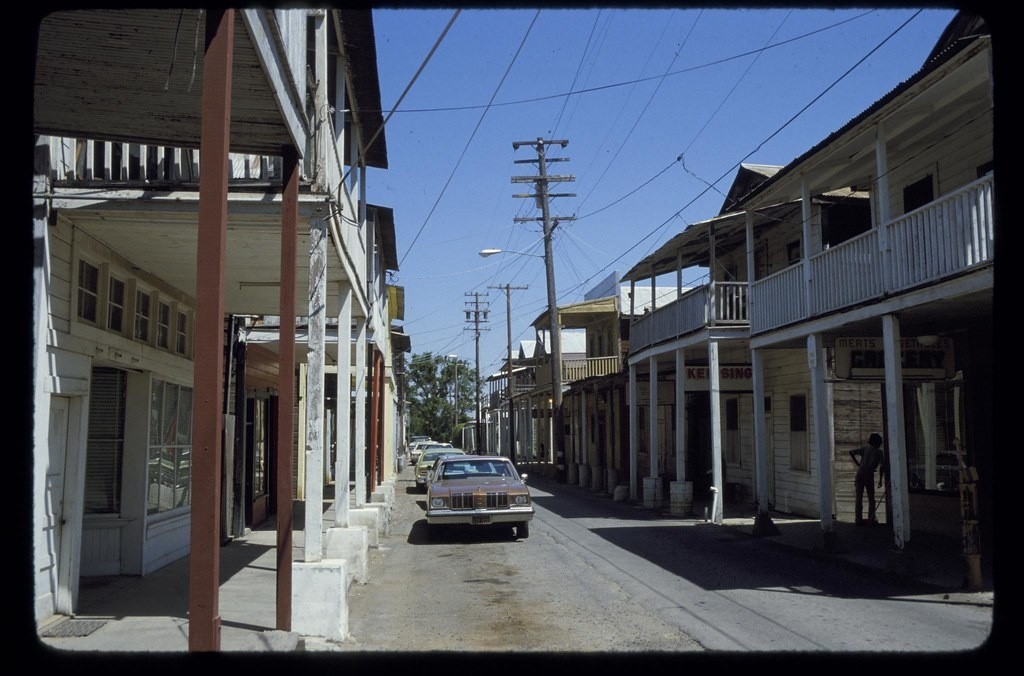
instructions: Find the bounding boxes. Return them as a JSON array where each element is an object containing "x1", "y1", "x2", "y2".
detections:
[
  {"x1": 415, "y1": 449, "x2": 467, "y2": 488},
  {"x1": 426, "y1": 454, "x2": 536, "y2": 539},
  {"x1": 408, "y1": 434, "x2": 453, "y2": 471}
]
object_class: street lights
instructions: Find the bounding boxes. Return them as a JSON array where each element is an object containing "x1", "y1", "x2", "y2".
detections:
[
  {"x1": 447, "y1": 353, "x2": 459, "y2": 429},
  {"x1": 478, "y1": 248, "x2": 563, "y2": 399}
]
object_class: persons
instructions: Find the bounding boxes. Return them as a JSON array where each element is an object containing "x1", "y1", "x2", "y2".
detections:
[{"x1": 848, "y1": 432, "x2": 887, "y2": 530}]
